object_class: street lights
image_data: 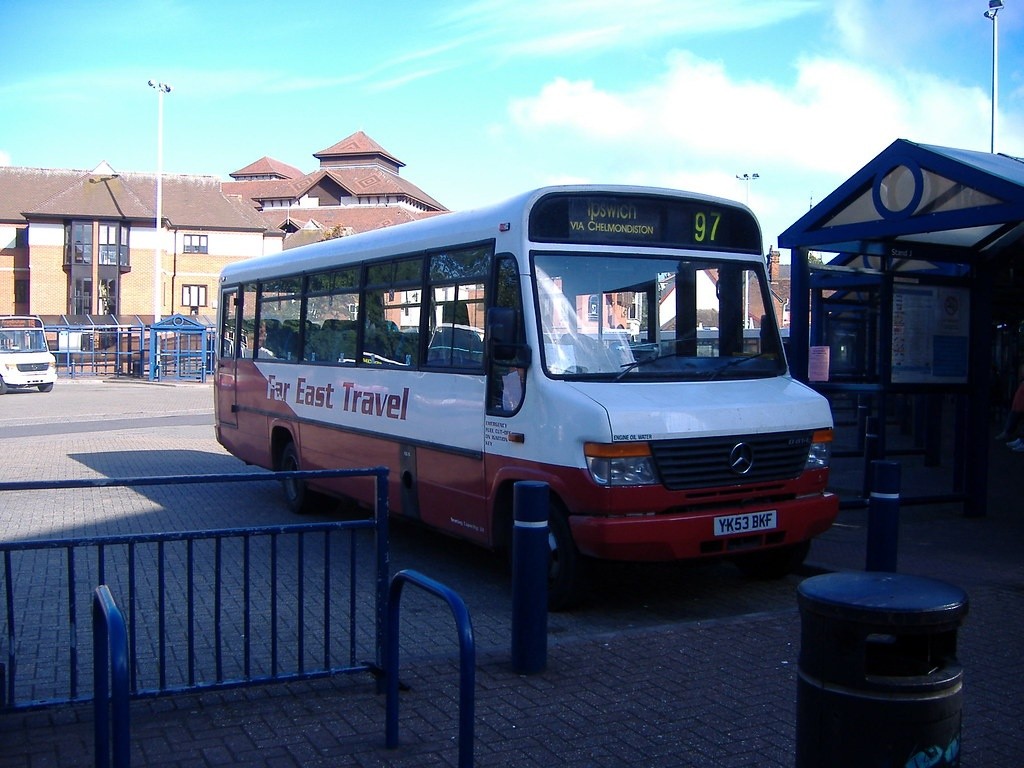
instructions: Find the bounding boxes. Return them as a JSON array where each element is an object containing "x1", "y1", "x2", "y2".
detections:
[
  {"x1": 983, "y1": 0, "x2": 1006, "y2": 153},
  {"x1": 736, "y1": 173, "x2": 759, "y2": 329},
  {"x1": 148, "y1": 80, "x2": 172, "y2": 379}
]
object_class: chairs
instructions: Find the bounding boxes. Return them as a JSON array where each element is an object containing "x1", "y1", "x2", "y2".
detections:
[{"x1": 227, "y1": 318, "x2": 608, "y2": 373}]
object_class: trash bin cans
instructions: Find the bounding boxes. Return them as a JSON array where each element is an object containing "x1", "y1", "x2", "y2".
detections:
[{"x1": 795, "y1": 571, "x2": 970, "y2": 768}]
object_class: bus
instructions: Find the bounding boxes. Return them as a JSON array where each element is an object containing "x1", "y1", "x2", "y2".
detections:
[
  {"x1": 214, "y1": 184, "x2": 840, "y2": 609},
  {"x1": 0, "y1": 316, "x2": 57, "y2": 394}
]
